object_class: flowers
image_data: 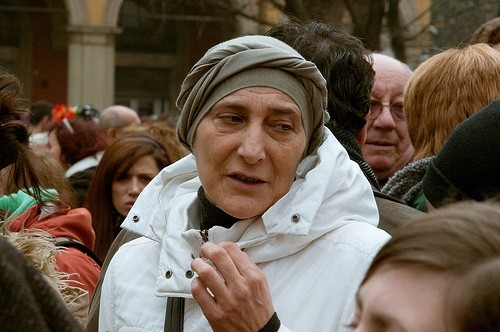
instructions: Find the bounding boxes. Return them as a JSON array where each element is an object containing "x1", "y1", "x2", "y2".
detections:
[{"x1": 51, "y1": 103, "x2": 75, "y2": 124}]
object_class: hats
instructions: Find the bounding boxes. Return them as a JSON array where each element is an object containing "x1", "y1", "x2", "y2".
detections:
[
  {"x1": 175, "y1": 34, "x2": 328, "y2": 161},
  {"x1": 422, "y1": 98, "x2": 500, "y2": 210}
]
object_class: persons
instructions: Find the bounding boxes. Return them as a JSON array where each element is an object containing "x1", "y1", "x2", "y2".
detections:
[{"x1": 0, "y1": 0, "x2": 500, "y2": 332}]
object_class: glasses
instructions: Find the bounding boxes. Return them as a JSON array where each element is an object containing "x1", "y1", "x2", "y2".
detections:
[{"x1": 366, "y1": 99, "x2": 407, "y2": 121}]
